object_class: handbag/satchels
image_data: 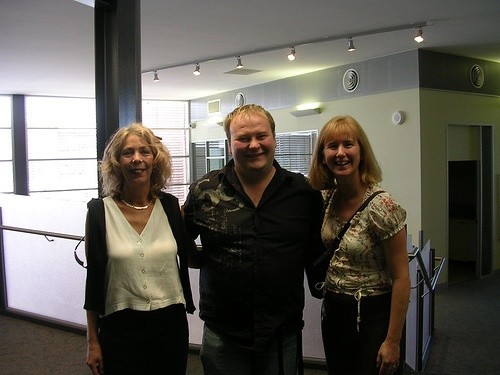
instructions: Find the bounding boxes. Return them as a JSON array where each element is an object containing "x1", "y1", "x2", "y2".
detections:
[{"x1": 308, "y1": 248, "x2": 335, "y2": 299}]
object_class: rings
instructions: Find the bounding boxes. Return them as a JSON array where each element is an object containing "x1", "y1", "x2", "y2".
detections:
[{"x1": 393, "y1": 365, "x2": 396, "y2": 367}]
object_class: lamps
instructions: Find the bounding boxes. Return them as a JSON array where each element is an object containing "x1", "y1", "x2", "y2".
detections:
[
  {"x1": 236, "y1": 55, "x2": 243, "y2": 69},
  {"x1": 192, "y1": 62, "x2": 201, "y2": 76},
  {"x1": 217, "y1": 122, "x2": 224, "y2": 126},
  {"x1": 288, "y1": 45, "x2": 296, "y2": 61},
  {"x1": 153, "y1": 70, "x2": 160, "y2": 83},
  {"x1": 413, "y1": 26, "x2": 424, "y2": 44},
  {"x1": 290, "y1": 107, "x2": 321, "y2": 118},
  {"x1": 344, "y1": 36, "x2": 356, "y2": 52}
]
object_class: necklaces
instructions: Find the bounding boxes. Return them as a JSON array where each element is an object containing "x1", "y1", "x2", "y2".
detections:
[{"x1": 118, "y1": 195, "x2": 154, "y2": 210}]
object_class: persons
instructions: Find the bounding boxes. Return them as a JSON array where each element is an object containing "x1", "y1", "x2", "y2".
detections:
[
  {"x1": 84, "y1": 122, "x2": 202, "y2": 375},
  {"x1": 309, "y1": 116, "x2": 411, "y2": 375},
  {"x1": 180, "y1": 104, "x2": 328, "y2": 375}
]
